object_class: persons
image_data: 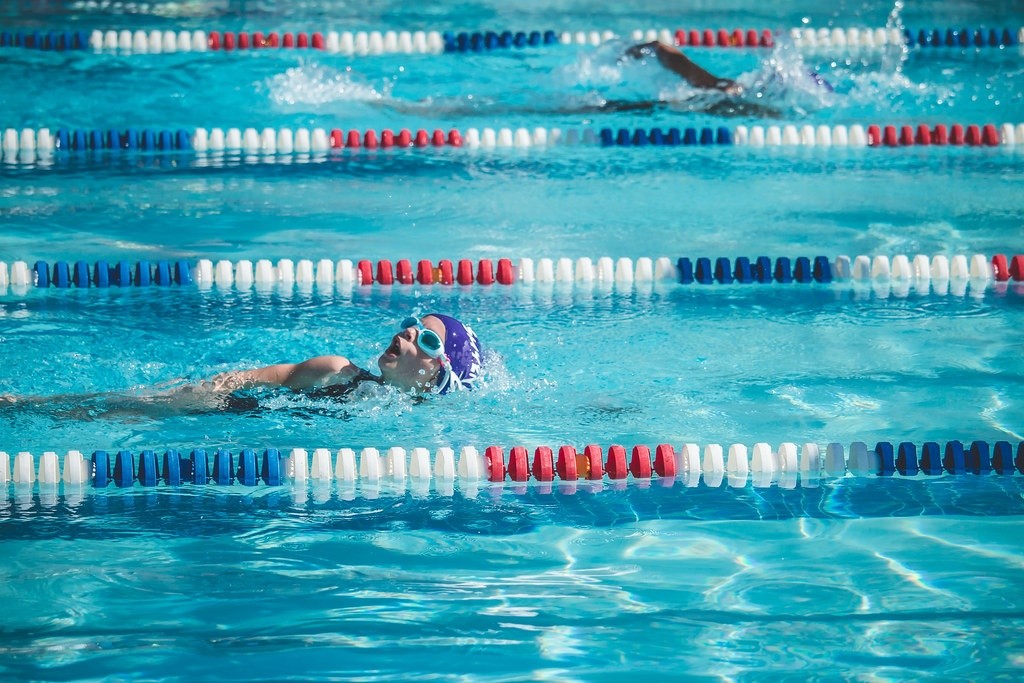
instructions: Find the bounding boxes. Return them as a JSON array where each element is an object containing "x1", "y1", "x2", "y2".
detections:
[
  {"x1": 367, "y1": 41, "x2": 835, "y2": 124},
  {"x1": 3, "y1": 313, "x2": 485, "y2": 427}
]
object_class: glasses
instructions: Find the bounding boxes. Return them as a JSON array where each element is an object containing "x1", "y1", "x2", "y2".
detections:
[{"x1": 402, "y1": 316, "x2": 463, "y2": 393}]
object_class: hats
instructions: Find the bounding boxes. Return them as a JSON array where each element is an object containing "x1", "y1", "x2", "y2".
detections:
[{"x1": 423, "y1": 313, "x2": 482, "y2": 395}]
